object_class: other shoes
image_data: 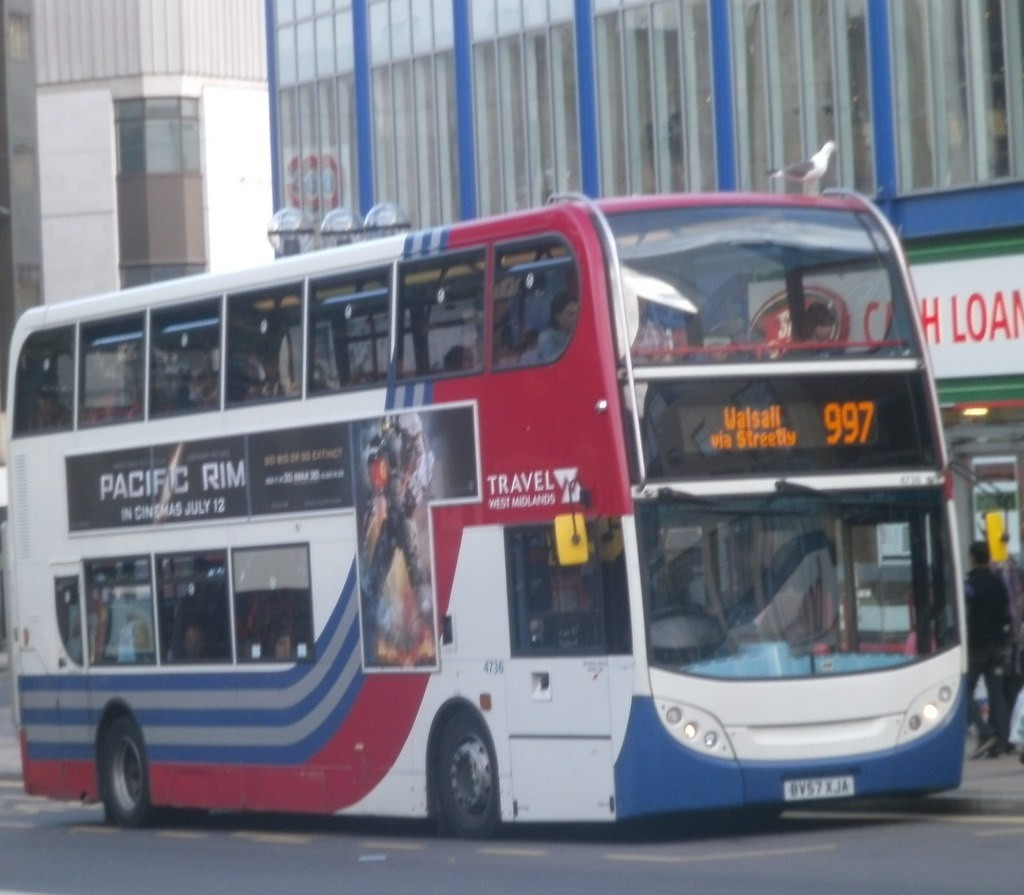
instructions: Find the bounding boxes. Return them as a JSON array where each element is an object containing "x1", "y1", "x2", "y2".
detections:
[
  {"x1": 971, "y1": 734, "x2": 996, "y2": 760},
  {"x1": 989, "y1": 743, "x2": 1015, "y2": 758}
]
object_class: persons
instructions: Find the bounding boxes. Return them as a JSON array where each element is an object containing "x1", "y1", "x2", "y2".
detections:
[
  {"x1": 784, "y1": 300, "x2": 840, "y2": 357},
  {"x1": 960, "y1": 540, "x2": 1012, "y2": 761},
  {"x1": 531, "y1": 292, "x2": 581, "y2": 358}
]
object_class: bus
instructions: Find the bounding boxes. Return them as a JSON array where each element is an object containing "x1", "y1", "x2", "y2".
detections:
[{"x1": 2, "y1": 164, "x2": 1009, "y2": 839}]
